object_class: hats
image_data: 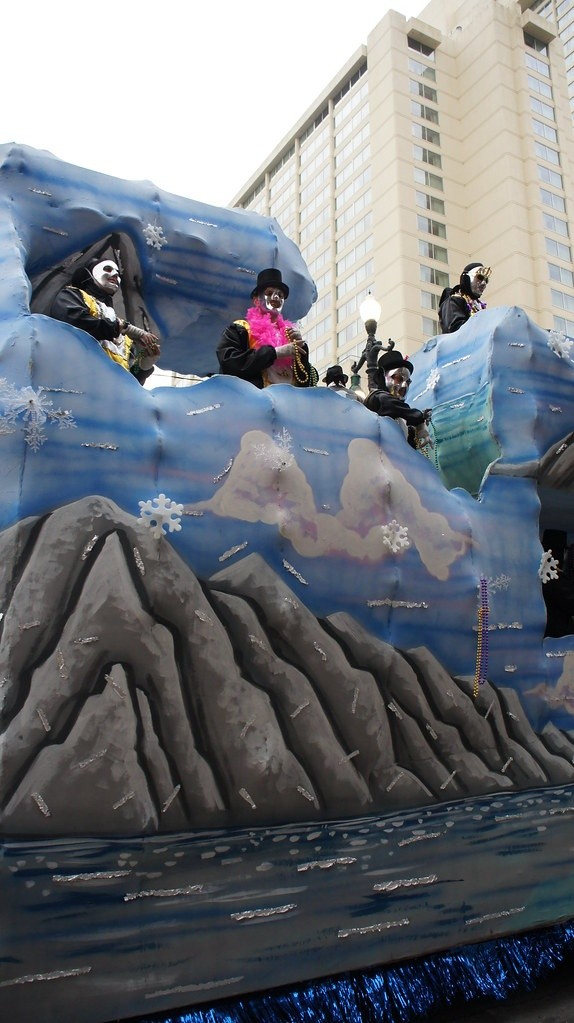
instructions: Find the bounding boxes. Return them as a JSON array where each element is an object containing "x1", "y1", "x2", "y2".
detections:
[
  {"x1": 322, "y1": 366, "x2": 350, "y2": 383},
  {"x1": 250, "y1": 267, "x2": 289, "y2": 300},
  {"x1": 373, "y1": 351, "x2": 414, "y2": 383}
]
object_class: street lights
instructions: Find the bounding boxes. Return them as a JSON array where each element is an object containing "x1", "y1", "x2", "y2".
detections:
[{"x1": 349, "y1": 292, "x2": 395, "y2": 404}]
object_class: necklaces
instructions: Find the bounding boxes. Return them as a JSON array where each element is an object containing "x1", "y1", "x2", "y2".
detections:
[
  {"x1": 306, "y1": 363, "x2": 318, "y2": 387},
  {"x1": 284, "y1": 326, "x2": 309, "y2": 386},
  {"x1": 131, "y1": 337, "x2": 158, "y2": 375},
  {"x1": 413, "y1": 412, "x2": 441, "y2": 470}
]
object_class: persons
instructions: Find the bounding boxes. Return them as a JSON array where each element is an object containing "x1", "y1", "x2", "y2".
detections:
[
  {"x1": 323, "y1": 366, "x2": 360, "y2": 401},
  {"x1": 217, "y1": 266, "x2": 318, "y2": 389},
  {"x1": 365, "y1": 350, "x2": 436, "y2": 453},
  {"x1": 51, "y1": 232, "x2": 161, "y2": 386}
]
